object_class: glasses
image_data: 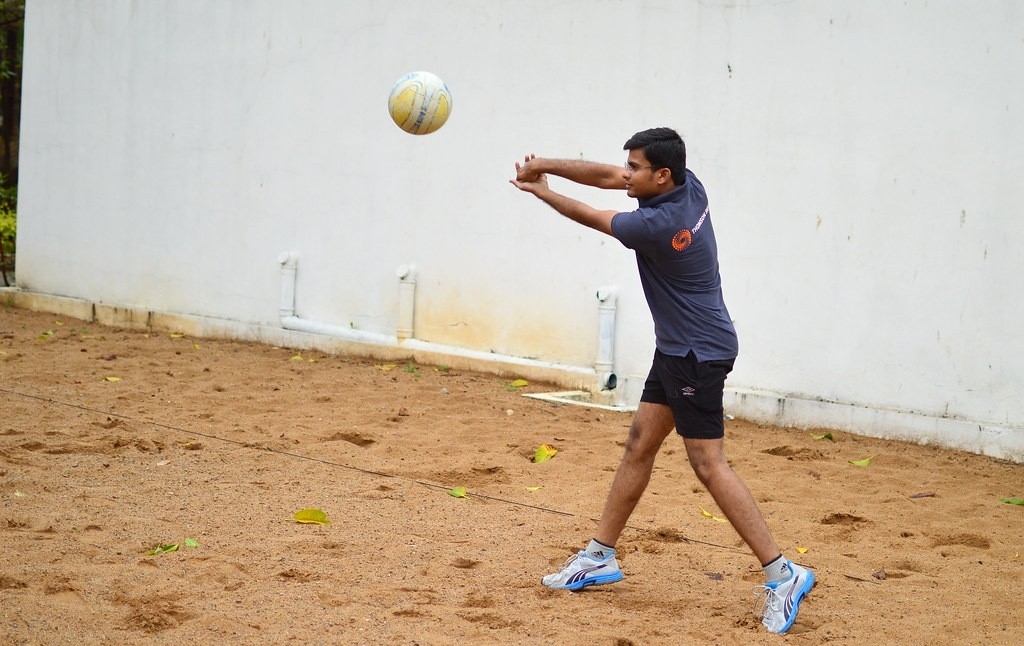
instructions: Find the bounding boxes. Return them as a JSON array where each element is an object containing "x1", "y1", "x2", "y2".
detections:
[{"x1": 623, "y1": 161, "x2": 664, "y2": 171}]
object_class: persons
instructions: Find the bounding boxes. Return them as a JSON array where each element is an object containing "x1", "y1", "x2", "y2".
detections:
[{"x1": 510, "y1": 126, "x2": 815, "y2": 634}]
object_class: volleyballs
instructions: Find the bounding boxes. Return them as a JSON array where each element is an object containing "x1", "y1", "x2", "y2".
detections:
[{"x1": 387, "y1": 69, "x2": 452, "y2": 135}]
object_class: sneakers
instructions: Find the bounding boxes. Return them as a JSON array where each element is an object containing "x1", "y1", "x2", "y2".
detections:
[
  {"x1": 541, "y1": 549, "x2": 624, "y2": 590},
  {"x1": 752, "y1": 559, "x2": 815, "y2": 636}
]
